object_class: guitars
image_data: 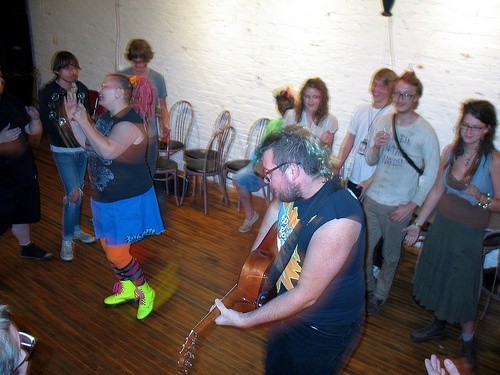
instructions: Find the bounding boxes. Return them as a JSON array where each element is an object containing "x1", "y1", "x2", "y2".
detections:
[{"x1": 176, "y1": 219, "x2": 279, "y2": 375}]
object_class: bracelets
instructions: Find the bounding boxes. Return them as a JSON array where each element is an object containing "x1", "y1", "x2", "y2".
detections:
[
  {"x1": 414, "y1": 222, "x2": 421, "y2": 228},
  {"x1": 478, "y1": 193, "x2": 492, "y2": 209}
]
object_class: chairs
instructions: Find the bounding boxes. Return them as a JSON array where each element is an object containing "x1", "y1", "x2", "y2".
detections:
[
  {"x1": 477, "y1": 232, "x2": 500, "y2": 320},
  {"x1": 151, "y1": 100, "x2": 236, "y2": 216},
  {"x1": 219, "y1": 118, "x2": 271, "y2": 213}
]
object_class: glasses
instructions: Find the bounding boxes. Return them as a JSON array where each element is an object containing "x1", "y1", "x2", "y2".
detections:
[
  {"x1": 12, "y1": 331, "x2": 39, "y2": 375},
  {"x1": 260, "y1": 159, "x2": 301, "y2": 182},
  {"x1": 391, "y1": 91, "x2": 416, "y2": 101},
  {"x1": 459, "y1": 121, "x2": 485, "y2": 132}
]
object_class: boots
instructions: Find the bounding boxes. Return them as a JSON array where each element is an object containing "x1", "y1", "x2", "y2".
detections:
[
  {"x1": 103, "y1": 280, "x2": 139, "y2": 306},
  {"x1": 135, "y1": 281, "x2": 156, "y2": 319}
]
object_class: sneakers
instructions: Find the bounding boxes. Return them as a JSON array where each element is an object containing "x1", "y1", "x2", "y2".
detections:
[
  {"x1": 61, "y1": 239, "x2": 73, "y2": 261},
  {"x1": 73, "y1": 229, "x2": 95, "y2": 244}
]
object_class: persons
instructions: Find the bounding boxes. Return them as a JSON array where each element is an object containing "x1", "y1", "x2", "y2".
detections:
[
  {"x1": 333, "y1": 69, "x2": 398, "y2": 281},
  {"x1": 232, "y1": 90, "x2": 295, "y2": 234},
  {"x1": 401, "y1": 100, "x2": 500, "y2": 368},
  {"x1": 208, "y1": 125, "x2": 367, "y2": 375},
  {"x1": 121, "y1": 39, "x2": 170, "y2": 178},
  {"x1": 38, "y1": 51, "x2": 96, "y2": 261},
  {"x1": 284, "y1": 78, "x2": 338, "y2": 151},
  {"x1": 0, "y1": 72, "x2": 53, "y2": 260},
  {"x1": 63, "y1": 73, "x2": 165, "y2": 319},
  {"x1": 362, "y1": 70, "x2": 440, "y2": 314},
  {"x1": 0, "y1": 304, "x2": 38, "y2": 375}
]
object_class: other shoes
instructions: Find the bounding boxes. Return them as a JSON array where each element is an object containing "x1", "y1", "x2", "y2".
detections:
[
  {"x1": 366, "y1": 295, "x2": 385, "y2": 315},
  {"x1": 20, "y1": 244, "x2": 52, "y2": 261},
  {"x1": 461, "y1": 334, "x2": 478, "y2": 370},
  {"x1": 373, "y1": 266, "x2": 380, "y2": 281},
  {"x1": 412, "y1": 321, "x2": 446, "y2": 342},
  {"x1": 239, "y1": 210, "x2": 259, "y2": 233}
]
